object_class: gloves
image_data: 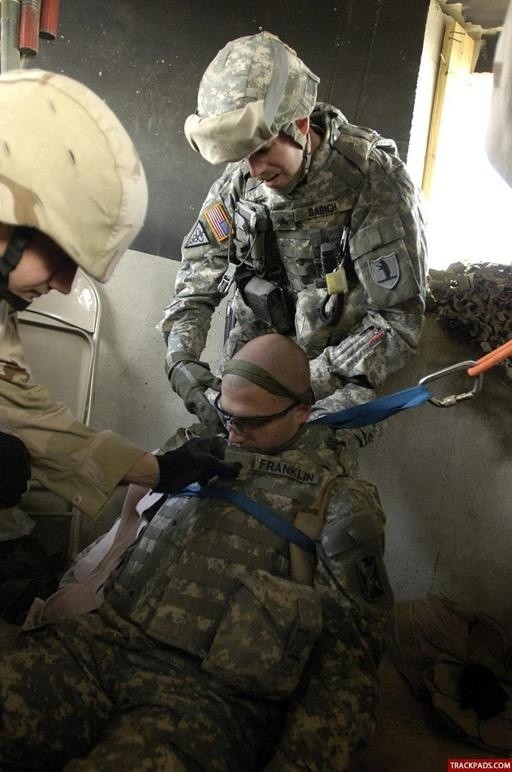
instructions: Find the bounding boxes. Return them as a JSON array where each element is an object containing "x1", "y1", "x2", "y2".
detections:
[
  {"x1": 151, "y1": 435, "x2": 242, "y2": 492},
  {"x1": 1, "y1": 432, "x2": 33, "y2": 504},
  {"x1": 163, "y1": 353, "x2": 228, "y2": 438}
]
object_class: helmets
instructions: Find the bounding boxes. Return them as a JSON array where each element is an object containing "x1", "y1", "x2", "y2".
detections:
[
  {"x1": 184, "y1": 32, "x2": 320, "y2": 164},
  {"x1": 0, "y1": 68, "x2": 149, "y2": 284}
]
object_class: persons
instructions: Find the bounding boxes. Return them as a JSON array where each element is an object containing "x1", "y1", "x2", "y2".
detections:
[
  {"x1": 0, "y1": 70, "x2": 243, "y2": 624},
  {"x1": 0, "y1": 334, "x2": 396, "y2": 772},
  {"x1": 159, "y1": 30, "x2": 428, "y2": 480}
]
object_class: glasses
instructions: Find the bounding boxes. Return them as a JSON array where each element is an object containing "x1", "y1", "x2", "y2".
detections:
[{"x1": 212, "y1": 388, "x2": 296, "y2": 431}]
